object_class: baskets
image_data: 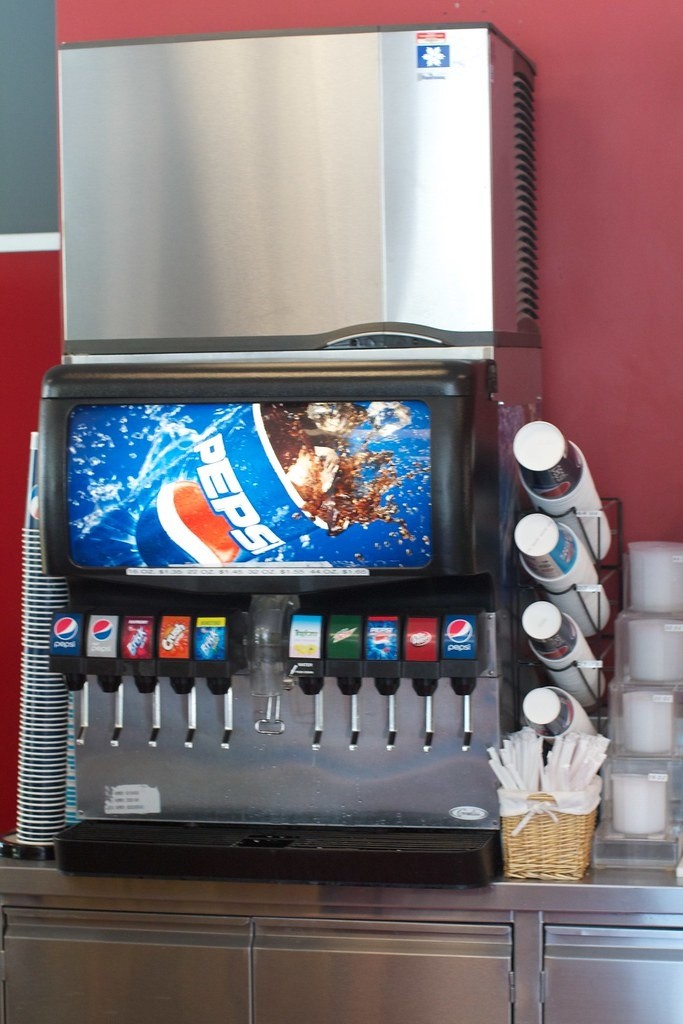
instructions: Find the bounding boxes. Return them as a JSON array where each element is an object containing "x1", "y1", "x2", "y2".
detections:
[{"x1": 500, "y1": 774, "x2": 602, "y2": 878}]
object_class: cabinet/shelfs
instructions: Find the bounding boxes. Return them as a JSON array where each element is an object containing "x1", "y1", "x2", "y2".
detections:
[
  {"x1": 0, "y1": 894, "x2": 517, "y2": 1024},
  {"x1": 539, "y1": 911, "x2": 683, "y2": 1024}
]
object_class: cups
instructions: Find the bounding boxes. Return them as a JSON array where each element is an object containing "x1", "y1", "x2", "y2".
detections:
[
  {"x1": 628, "y1": 619, "x2": 683, "y2": 682},
  {"x1": 17, "y1": 433, "x2": 81, "y2": 846},
  {"x1": 513, "y1": 420, "x2": 610, "y2": 748},
  {"x1": 611, "y1": 774, "x2": 667, "y2": 833},
  {"x1": 622, "y1": 692, "x2": 672, "y2": 753},
  {"x1": 135, "y1": 404, "x2": 348, "y2": 567},
  {"x1": 628, "y1": 541, "x2": 683, "y2": 613}
]
